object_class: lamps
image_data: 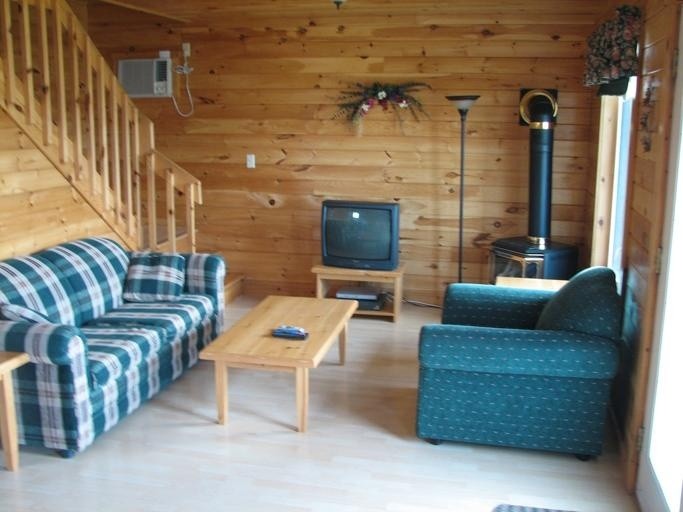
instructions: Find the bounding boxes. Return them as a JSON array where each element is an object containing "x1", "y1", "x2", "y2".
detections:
[{"x1": 443, "y1": 96, "x2": 480, "y2": 283}]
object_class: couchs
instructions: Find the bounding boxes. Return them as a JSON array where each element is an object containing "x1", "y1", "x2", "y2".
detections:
[{"x1": 0, "y1": 238, "x2": 226, "y2": 458}]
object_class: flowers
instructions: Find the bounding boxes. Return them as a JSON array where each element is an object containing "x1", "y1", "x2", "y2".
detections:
[{"x1": 328, "y1": 84, "x2": 436, "y2": 137}]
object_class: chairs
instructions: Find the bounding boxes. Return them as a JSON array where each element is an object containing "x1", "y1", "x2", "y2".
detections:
[{"x1": 417, "y1": 266, "x2": 623, "y2": 462}]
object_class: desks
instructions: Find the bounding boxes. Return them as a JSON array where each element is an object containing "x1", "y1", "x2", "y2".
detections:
[{"x1": 310, "y1": 262, "x2": 403, "y2": 322}]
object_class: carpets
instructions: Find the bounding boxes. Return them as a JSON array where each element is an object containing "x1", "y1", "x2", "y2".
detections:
[{"x1": 491, "y1": 504, "x2": 578, "y2": 512}]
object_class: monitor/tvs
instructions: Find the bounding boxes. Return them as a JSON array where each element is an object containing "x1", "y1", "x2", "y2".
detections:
[{"x1": 320, "y1": 200, "x2": 398, "y2": 270}]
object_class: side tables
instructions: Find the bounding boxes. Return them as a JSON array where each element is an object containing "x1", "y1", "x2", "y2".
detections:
[
  {"x1": 0, "y1": 351, "x2": 30, "y2": 471},
  {"x1": 495, "y1": 275, "x2": 570, "y2": 292}
]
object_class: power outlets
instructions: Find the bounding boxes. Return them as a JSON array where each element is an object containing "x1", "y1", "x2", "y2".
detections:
[{"x1": 182, "y1": 42, "x2": 190, "y2": 57}]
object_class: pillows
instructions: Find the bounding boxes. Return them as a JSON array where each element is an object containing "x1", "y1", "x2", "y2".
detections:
[
  {"x1": 534, "y1": 266, "x2": 623, "y2": 344},
  {"x1": 122, "y1": 252, "x2": 187, "y2": 304},
  {"x1": 0, "y1": 301, "x2": 54, "y2": 325}
]
